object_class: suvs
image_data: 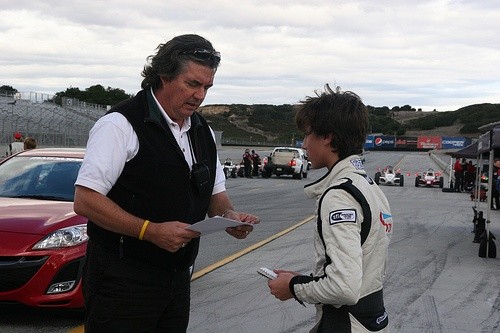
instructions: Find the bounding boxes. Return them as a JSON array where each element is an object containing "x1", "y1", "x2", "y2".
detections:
[{"x1": 261, "y1": 146, "x2": 310, "y2": 179}]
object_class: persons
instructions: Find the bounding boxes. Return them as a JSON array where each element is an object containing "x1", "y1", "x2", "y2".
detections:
[
  {"x1": 454, "y1": 157, "x2": 463, "y2": 192},
  {"x1": 460, "y1": 158, "x2": 468, "y2": 190},
  {"x1": 491, "y1": 160, "x2": 500, "y2": 209},
  {"x1": 468, "y1": 160, "x2": 476, "y2": 189},
  {"x1": 268, "y1": 83, "x2": 393, "y2": 332},
  {"x1": 74, "y1": 34, "x2": 262, "y2": 332},
  {"x1": 251, "y1": 150, "x2": 261, "y2": 177},
  {"x1": 243, "y1": 149, "x2": 252, "y2": 178}
]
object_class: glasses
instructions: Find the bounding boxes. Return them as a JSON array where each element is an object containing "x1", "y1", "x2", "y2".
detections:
[{"x1": 181, "y1": 49, "x2": 222, "y2": 62}]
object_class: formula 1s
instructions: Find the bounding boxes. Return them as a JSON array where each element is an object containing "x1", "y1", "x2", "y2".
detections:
[
  {"x1": 414, "y1": 167, "x2": 443, "y2": 188},
  {"x1": 374, "y1": 165, "x2": 404, "y2": 187}
]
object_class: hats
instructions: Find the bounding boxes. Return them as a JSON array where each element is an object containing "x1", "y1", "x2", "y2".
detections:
[{"x1": 245, "y1": 149, "x2": 250, "y2": 152}]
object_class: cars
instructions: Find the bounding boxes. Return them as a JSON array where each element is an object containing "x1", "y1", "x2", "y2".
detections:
[{"x1": 0, "y1": 148, "x2": 92, "y2": 323}]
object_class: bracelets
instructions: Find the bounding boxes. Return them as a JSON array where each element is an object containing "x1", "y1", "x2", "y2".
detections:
[{"x1": 139, "y1": 219, "x2": 150, "y2": 240}]
